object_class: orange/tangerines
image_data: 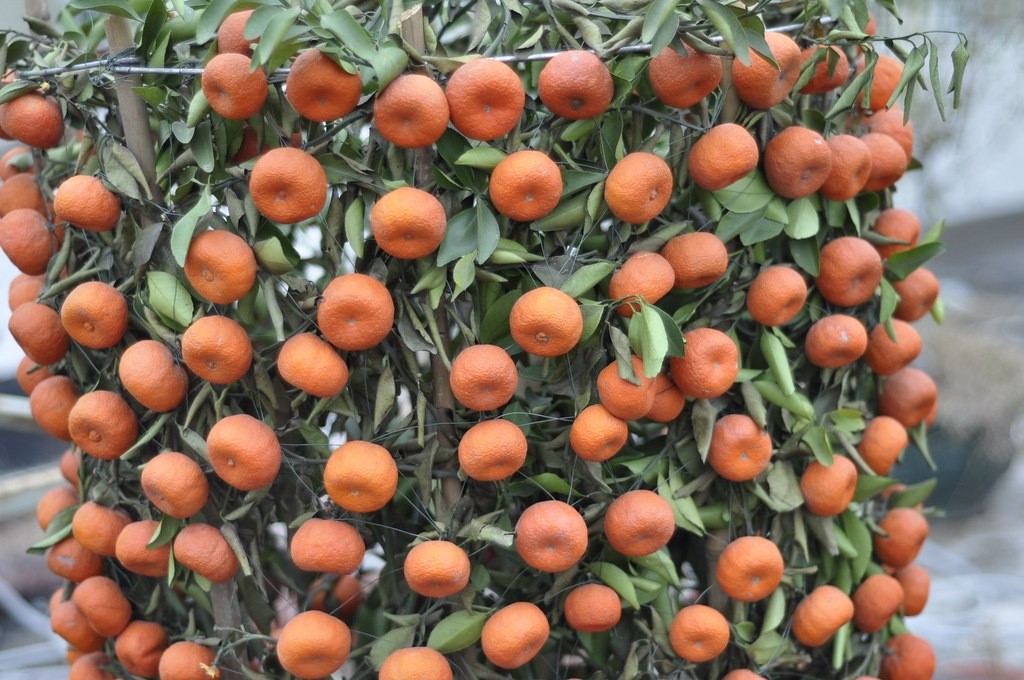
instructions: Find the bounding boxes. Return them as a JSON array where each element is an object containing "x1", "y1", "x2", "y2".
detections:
[{"x1": 1, "y1": 6, "x2": 934, "y2": 680}]
